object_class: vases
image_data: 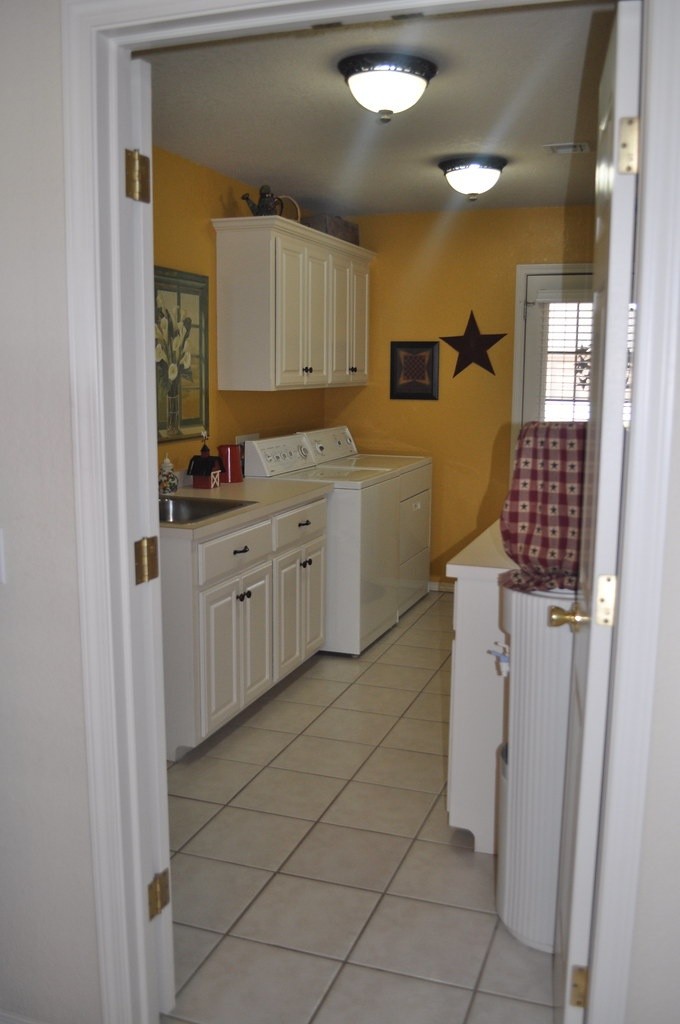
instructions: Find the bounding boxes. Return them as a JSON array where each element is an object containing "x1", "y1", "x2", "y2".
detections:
[{"x1": 167, "y1": 395, "x2": 181, "y2": 435}]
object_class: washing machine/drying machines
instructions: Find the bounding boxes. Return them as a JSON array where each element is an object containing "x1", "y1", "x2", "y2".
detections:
[{"x1": 241, "y1": 423, "x2": 434, "y2": 660}]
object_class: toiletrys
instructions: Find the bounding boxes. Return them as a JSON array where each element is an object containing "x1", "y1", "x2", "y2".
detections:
[{"x1": 158, "y1": 452, "x2": 179, "y2": 495}]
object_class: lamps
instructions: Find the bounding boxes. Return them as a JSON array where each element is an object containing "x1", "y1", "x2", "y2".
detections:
[
  {"x1": 438, "y1": 154, "x2": 508, "y2": 201},
  {"x1": 337, "y1": 53, "x2": 439, "y2": 123}
]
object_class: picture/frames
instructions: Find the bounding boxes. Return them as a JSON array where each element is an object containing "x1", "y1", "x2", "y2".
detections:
[
  {"x1": 390, "y1": 341, "x2": 439, "y2": 400},
  {"x1": 154, "y1": 264, "x2": 211, "y2": 442}
]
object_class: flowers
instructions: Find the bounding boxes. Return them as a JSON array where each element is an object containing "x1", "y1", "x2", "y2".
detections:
[{"x1": 155, "y1": 307, "x2": 194, "y2": 397}]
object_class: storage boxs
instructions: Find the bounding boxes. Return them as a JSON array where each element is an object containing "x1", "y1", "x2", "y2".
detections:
[{"x1": 300, "y1": 214, "x2": 360, "y2": 246}]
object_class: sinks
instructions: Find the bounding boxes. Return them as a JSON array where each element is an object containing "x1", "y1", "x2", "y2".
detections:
[{"x1": 159, "y1": 494, "x2": 260, "y2": 525}]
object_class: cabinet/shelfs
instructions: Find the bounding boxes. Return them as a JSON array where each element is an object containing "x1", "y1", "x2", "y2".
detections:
[
  {"x1": 445, "y1": 515, "x2": 519, "y2": 855},
  {"x1": 211, "y1": 215, "x2": 379, "y2": 391},
  {"x1": 160, "y1": 482, "x2": 333, "y2": 762}
]
